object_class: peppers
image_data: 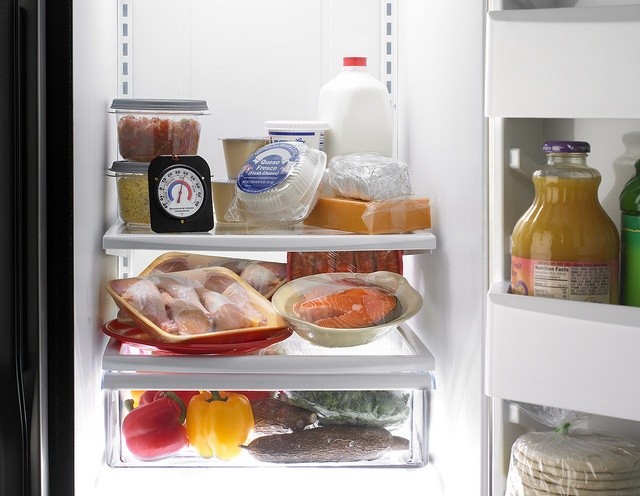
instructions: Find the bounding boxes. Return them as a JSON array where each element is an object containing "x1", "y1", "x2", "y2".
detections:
[
  {"x1": 187, "y1": 391, "x2": 274, "y2": 461},
  {"x1": 122, "y1": 391, "x2": 200, "y2": 462}
]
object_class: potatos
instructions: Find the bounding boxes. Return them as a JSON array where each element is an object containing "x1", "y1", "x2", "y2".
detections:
[
  {"x1": 239, "y1": 426, "x2": 410, "y2": 464},
  {"x1": 250, "y1": 398, "x2": 318, "y2": 432}
]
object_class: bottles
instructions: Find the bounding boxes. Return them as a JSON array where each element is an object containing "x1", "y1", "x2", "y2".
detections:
[
  {"x1": 619, "y1": 158, "x2": 640, "y2": 306},
  {"x1": 509, "y1": 140, "x2": 620, "y2": 305}
]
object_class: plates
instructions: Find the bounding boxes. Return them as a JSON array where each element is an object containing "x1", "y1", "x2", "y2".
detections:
[
  {"x1": 104, "y1": 265, "x2": 290, "y2": 345},
  {"x1": 117, "y1": 251, "x2": 287, "y2": 326},
  {"x1": 270, "y1": 270, "x2": 424, "y2": 348},
  {"x1": 102, "y1": 316, "x2": 293, "y2": 356}
]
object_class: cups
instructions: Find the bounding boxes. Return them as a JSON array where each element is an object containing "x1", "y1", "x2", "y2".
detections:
[{"x1": 264, "y1": 120, "x2": 329, "y2": 151}]
